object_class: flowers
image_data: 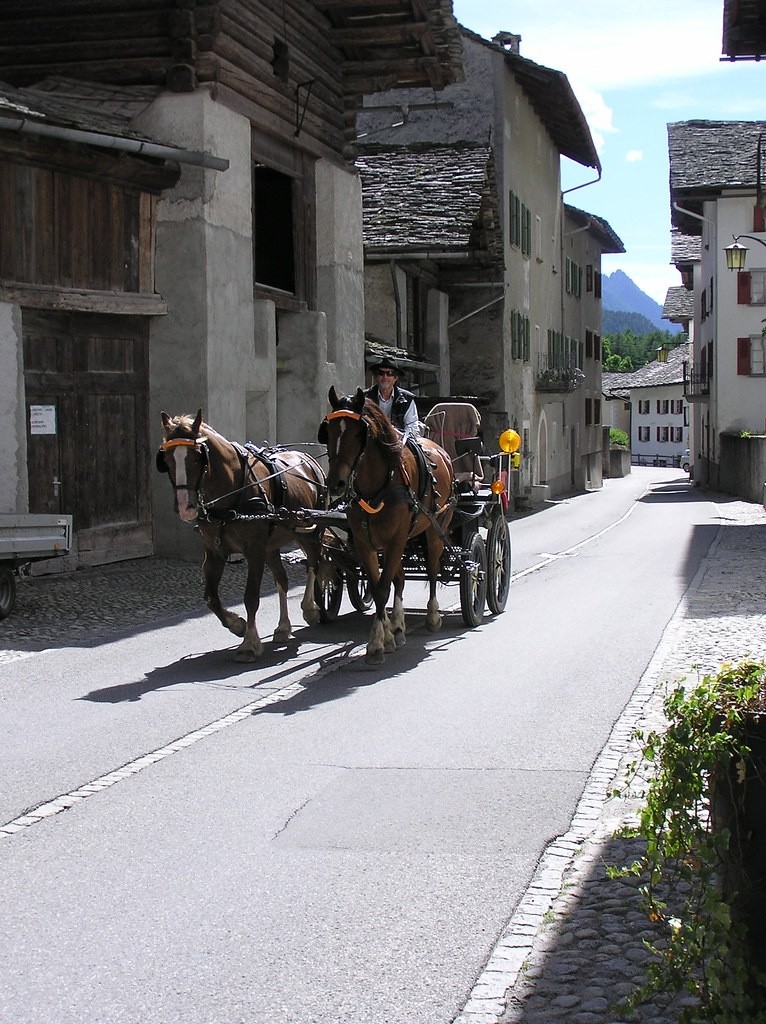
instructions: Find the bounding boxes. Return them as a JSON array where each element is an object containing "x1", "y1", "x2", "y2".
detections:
[{"x1": 536, "y1": 366, "x2": 585, "y2": 390}]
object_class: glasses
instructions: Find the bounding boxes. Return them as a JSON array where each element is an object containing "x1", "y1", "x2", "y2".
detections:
[{"x1": 374, "y1": 369, "x2": 398, "y2": 377}]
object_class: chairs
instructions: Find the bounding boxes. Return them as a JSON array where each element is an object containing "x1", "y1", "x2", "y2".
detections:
[{"x1": 419, "y1": 401, "x2": 485, "y2": 497}]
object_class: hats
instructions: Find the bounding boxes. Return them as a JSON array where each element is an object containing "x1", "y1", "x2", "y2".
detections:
[{"x1": 370, "y1": 356, "x2": 405, "y2": 377}]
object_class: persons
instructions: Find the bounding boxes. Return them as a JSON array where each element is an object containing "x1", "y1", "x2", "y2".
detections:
[{"x1": 362, "y1": 356, "x2": 420, "y2": 436}]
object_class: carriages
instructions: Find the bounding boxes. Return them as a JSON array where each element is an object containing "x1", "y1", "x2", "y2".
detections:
[{"x1": 153, "y1": 392, "x2": 527, "y2": 670}]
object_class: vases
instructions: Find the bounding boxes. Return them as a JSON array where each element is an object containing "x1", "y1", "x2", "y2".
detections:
[{"x1": 535, "y1": 388, "x2": 571, "y2": 404}]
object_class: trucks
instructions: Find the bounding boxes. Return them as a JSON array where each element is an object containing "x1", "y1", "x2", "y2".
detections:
[{"x1": 0, "y1": 514, "x2": 72, "y2": 619}]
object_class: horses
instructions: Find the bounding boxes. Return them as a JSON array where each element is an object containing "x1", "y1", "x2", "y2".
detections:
[
  {"x1": 318, "y1": 384, "x2": 456, "y2": 665},
  {"x1": 156, "y1": 407, "x2": 328, "y2": 664}
]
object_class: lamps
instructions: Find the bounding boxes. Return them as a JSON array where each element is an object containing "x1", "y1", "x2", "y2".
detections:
[{"x1": 722, "y1": 233, "x2": 766, "y2": 272}]
